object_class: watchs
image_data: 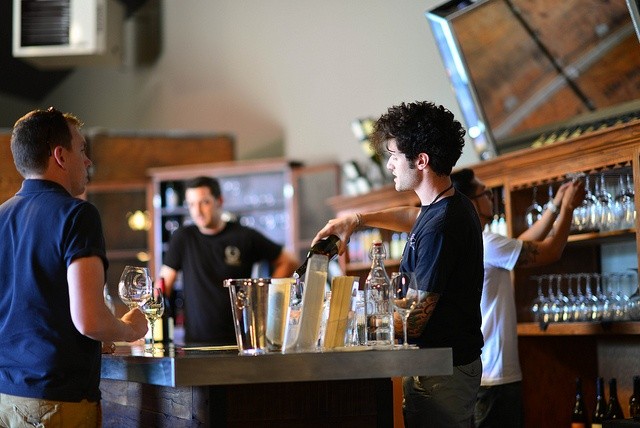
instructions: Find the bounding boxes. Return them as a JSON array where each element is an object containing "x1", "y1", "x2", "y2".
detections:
[{"x1": 545, "y1": 201, "x2": 559, "y2": 217}]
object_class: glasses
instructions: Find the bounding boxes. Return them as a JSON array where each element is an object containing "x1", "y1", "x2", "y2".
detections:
[
  {"x1": 469, "y1": 188, "x2": 493, "y2": 199},
  {"x1": 183, "y1": 199, "x2": 218, "y2": 210}
]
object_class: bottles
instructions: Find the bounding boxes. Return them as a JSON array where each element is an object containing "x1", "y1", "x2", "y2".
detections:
[
  {"x1": 627, "y1": 376, "x2": 639, "y2": 421},
  {"x1": 363, "y1": 240, "x2": 394, "y2": 347},
  {"x1": 605, "y1": 377, "x2": 624, "y2": 420},
  {"x1": 292, "y1": 234, "x2": 350, "y2": 280},
  {"x1": 570, "y1": 377, "x2": 587, "y2": 428},
  {"x1": 591, "y1": 376, "x2": 608, "y2": 428}
]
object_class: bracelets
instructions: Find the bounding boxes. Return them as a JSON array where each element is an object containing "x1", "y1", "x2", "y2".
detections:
[{"x1": 354, "y1": 211, "x2": 363, "y2": 229}]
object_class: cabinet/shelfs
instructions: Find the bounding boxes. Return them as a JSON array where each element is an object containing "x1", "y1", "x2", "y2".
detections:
[
  {"x1": 144, "y1": 157, "x2": 339, "y2": 325},
  {"x1": 325, "y1": 121, "x2": 637, "y2": 427}
]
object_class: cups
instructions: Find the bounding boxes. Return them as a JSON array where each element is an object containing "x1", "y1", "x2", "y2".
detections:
[{"x1": 223, "y1": 278, "x2": 272, "y2": 355}]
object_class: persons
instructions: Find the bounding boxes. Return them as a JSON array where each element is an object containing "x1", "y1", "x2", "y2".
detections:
[
  {"x1": 1, "y1": 106, "x2": 151, "y2": 428},
  {"x1": 450, "y1": 168, "x2": 588, "y2": 428},
  {"x1": 152, "y1": 175, "x2": 303, "y2": 346},
  {"x1": 309, "y1": 101, "x2": 486, "y2": 428}
]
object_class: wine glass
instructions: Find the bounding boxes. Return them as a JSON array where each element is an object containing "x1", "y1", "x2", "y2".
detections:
[
  {"x1": 139, "y1": 288, "x2": 168, "y2": 353},
  {"x1": 118, "y1": 265, "x2": 149, "y2": 311},
  {"x1": 528, "y1": 269, "x2": 640, "y2": 321},
  {"x1": 521, "y1": 171, "x2": 635, "y2": 234},
  {"x1": 390, "y1": 271, "x2": 421, "y2": 349}
]
